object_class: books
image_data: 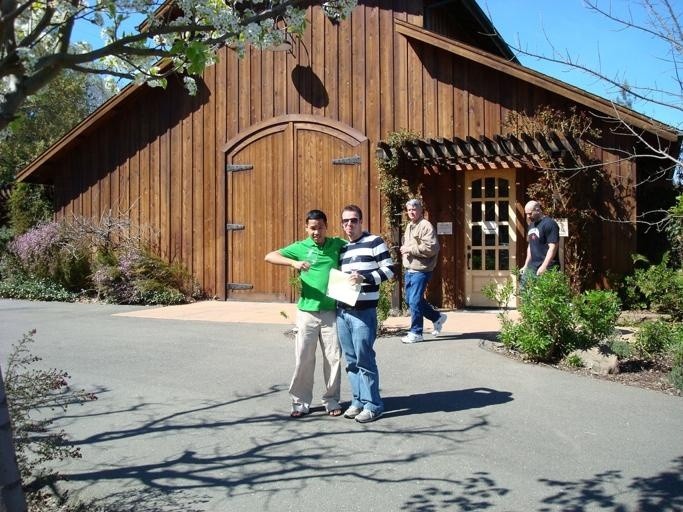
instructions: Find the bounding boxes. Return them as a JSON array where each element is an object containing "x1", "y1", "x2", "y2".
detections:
[{"x1": 326, "y1": 268, "x2": 362, "y2": 308}]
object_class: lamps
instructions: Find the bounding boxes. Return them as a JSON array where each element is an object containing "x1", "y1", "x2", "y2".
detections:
[{"x1": 265, "y1": 14, "x2": 297, "y2": 60}]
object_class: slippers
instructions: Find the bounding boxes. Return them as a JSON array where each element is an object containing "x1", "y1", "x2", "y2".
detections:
[
  {"x1": 328, "y1": 408, "x2": 342, "y2": 416},
  {"x1": 290, "y1": 409, "x2": 304, "y2": 417}
]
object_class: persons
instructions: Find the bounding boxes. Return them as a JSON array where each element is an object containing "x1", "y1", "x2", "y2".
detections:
[
  {"x1": 335, "y1": 205, "x2": 396, "y2": 423},
  {"x1": 398, "y1": 199, "x2": 447, "y2": 344},
  {"x1": 264, "y1": 209, "x2": 352, "y2": 418},
  {"x1": 519, "y1": 200, "x2": 560, "y2": 295}
]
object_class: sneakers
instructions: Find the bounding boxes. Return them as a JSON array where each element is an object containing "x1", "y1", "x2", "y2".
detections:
[
  {"x1": 343, "y1": 404, "x2": 362, "y2": 419},
  {"x1": 355, "y1": 408, "x2": 384, "y2": 424},
  {"x1": 431, "y1": 313, "x2": 448, "y2": 337},
  {"x1": 401, "y1": 331, "x2": 424, "y2": 344}
]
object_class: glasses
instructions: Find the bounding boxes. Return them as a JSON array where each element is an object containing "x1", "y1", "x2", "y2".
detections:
[{"x1": 341, "y1": 217, "x2": 360, "y2": 225}]
